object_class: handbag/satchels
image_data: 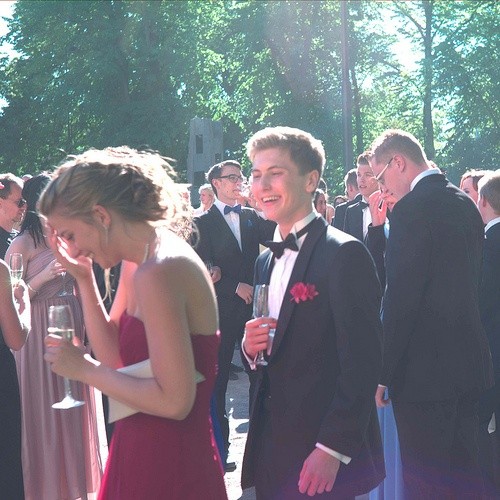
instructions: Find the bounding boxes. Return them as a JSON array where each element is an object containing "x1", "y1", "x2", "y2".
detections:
[{"x1": 109, "y1": 355, "x2": 205, "y2": 424}]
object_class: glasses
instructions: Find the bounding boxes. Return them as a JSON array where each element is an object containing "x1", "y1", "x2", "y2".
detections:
[
  {"x1": 373, "y1": 155, "x2": 396, "y2": 186},
  {"x1": 216, "y1": 173, "x2": 244, "y2": 184},
  {"x1": 4, "y1": 196, "x2": 27, "y2": 209}
]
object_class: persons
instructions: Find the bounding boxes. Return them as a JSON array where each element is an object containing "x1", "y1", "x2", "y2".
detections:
[
  {"x1": 331, "y1": 169, "x2": 362, "y2": 232},
  {"x1": 187, "y1": 160, "x2": 277, "y2": 474},
  {"x1": 241, "y1": 126, "x2": 386, "y2": 500},
  {"x1": 0, "y1": 258, "x2": 30, "y2": 500},
  {"x1": 313, "y1": 189, "x2": 327, "y2": 222},
  {"x1": 6, "y1": 172, "x2": 108, "y2": 500},
  {"x1": 334, "y1": 195, "x2": 345, "y2": 208},
  {"x1": 0, "y1": 173, "x2": 33, "y2": 261},
  {"x1": 237, "y1": 172, "x2": 264, "y2": 214},
  {"x1": 37, "y1": 144, "x2": 229, "y2": 500},
  {"x1": 363, "y1": 159, "x2": 438, "y2": 300},
  {"x1": 476, "y1": 168, "x2": 500, "y2": 500},
  {"x1": 367, "y1": 128, "x2": 500, "y2": 500},
  {"x1": 345, "y1": 151, "x2": 377, "y2": 243},
  {"x1": 191, "y1": 184, "x2": 215, "y2": 219},
  {"x1": 459, "y1": 169, "x2": 490, "y2": 205}
]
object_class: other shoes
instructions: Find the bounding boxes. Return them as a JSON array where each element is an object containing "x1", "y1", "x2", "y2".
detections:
[
  {"x1": 229, "y1": 363, "x2": 244, "y2": 371},
  {"x1": 226, "y1": 458, "x2": 236, "y2": 470},
  {"x1": 228, "y1": 372, "x2": 239, "y2": 380}
]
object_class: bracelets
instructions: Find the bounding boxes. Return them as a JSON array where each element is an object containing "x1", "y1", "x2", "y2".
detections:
[{"x1": 27, "y1": 283, "x2": 40, "y2": 293}]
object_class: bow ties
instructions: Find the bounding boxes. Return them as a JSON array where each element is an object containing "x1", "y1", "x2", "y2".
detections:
[
  {"x1": 264, "y1": 217, "x2": 317, "y2": 259},
  {"x1": 359, "y1": 201, "x2": 382, "y2": 209},
  {"x1": 224, "y1": 204, "x2": 241, "y2": 214}
]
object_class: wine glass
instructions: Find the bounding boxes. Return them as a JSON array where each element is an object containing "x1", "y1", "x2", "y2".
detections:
[
  {"x1": 47, "y1": 305, "x2": 86, "y2": 409},
  {"x1": 9, "y1": 252, "x2": 23, "y2": 303},
  {"x1": 252, "y1": 284, "x2": 276, "y2": 366}
]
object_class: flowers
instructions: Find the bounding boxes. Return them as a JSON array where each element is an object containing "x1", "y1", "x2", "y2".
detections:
[{"x1": 290, "y1": 282, "x2": 320, "y2": 304}]
